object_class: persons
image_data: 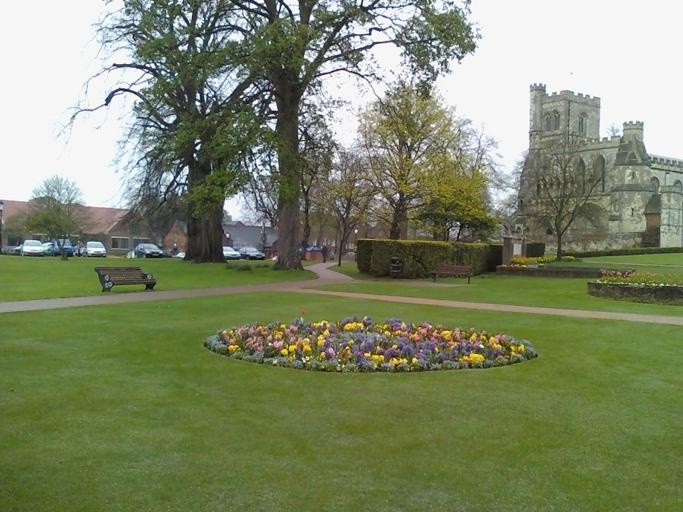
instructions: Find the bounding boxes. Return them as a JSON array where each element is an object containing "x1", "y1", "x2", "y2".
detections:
[{"x1": 321, "y1": 244, "x2": 328, "y2": 263}]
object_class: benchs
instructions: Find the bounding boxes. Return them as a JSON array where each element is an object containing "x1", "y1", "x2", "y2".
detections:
[
  {"x1": 94, "y1": 267, "x2": 157, "y2": 292},
  {"x1": 429, "y1": 265, "x2": 473, "y2": 284}
]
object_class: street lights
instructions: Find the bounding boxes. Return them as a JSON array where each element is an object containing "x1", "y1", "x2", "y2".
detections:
[
  {"x1": 260, "y1": 221, "x2": 266, "y2": 254},
  {"x1": 354, "y1": 227, "x2": 358, "y2": 253}
]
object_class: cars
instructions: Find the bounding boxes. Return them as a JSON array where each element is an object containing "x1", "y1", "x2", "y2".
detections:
[
  {"x1": 20, "y1": 239, "x2": 46, "y2": 257},
  {"x1": 132, "y1": 242, "x2": 166, "y2": 259},
  {"x1": 51, "y1": 238, "x2": 76, "y2": 258},
  {"x1": 237, "y1": 245, "x2": 267, "y2": 261},
  {"x1": 81, "y1": 240, "x2": 108, "y2": 258},
  {"x1": 222, "y1": 244, "x2": 243, "y2": 261}
]
